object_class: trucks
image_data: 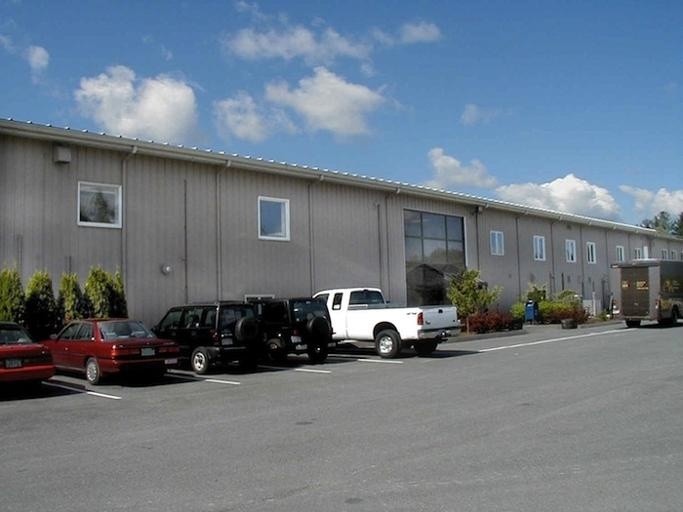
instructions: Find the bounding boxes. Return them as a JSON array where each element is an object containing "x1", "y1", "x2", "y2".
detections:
[{"x1": 607, "y1": 258, "x2": 683, "y2": 327}]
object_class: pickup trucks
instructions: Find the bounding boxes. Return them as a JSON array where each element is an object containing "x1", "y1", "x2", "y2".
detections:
[{"x1": 312, "y1": 287, "x2": 464, "y2": 358}]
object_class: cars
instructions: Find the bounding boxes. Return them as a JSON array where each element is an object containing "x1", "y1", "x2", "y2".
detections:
[
  {"x1": 39, "y1": 312, "x2": 183, "y2": 385},
  {"x1": 0, "y1": 321, "x2": 54, "y2": 390}
]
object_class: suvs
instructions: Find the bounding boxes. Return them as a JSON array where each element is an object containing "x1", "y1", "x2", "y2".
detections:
[
  {"x1": 238, "y1": 297, "x2": 334, "y2": 363},
  {"x1": 149, "y1": 300, "x2": 267, "y2": 375}
]
